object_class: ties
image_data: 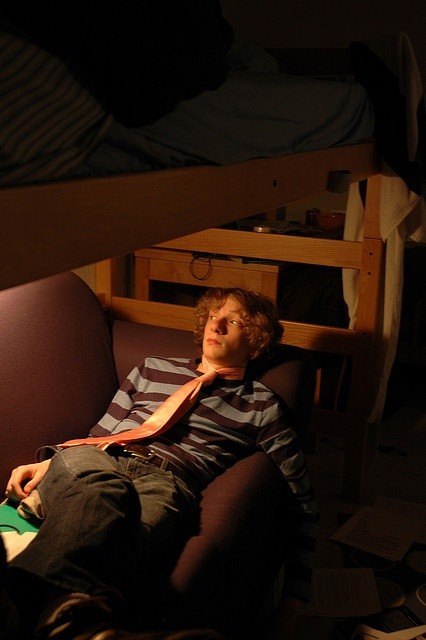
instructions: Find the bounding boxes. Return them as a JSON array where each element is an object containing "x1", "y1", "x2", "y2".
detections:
[{"x1": 55, "y1": 354, "x2": 247, "y2": 449}]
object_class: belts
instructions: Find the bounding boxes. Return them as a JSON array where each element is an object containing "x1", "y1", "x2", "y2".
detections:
[{"x1": 123, "y1": 443, "x2": 187, "y2": 482}]
